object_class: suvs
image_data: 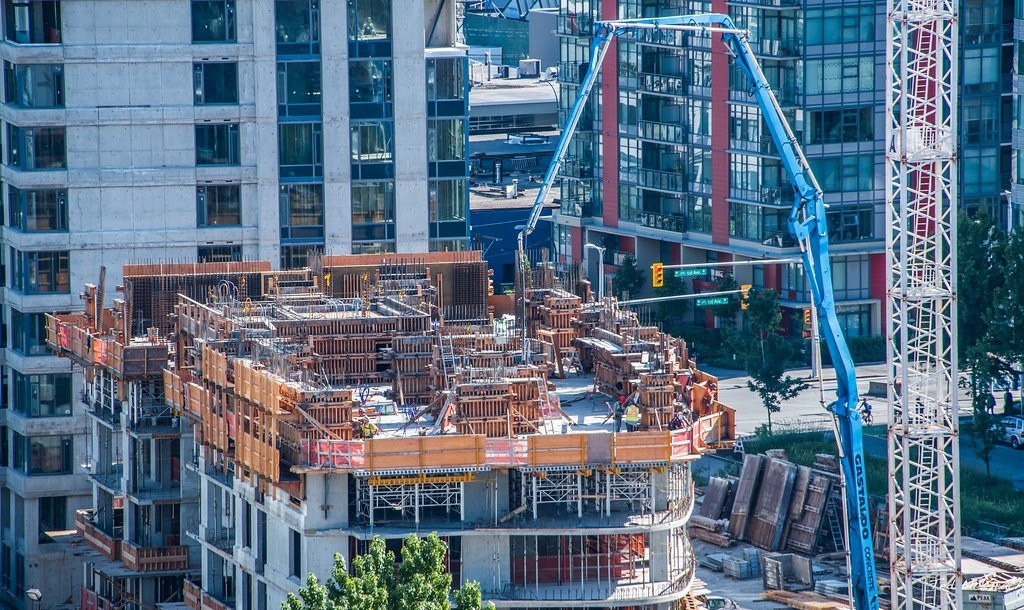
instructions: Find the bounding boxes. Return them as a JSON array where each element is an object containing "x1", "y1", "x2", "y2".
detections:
[{"x1": 986, "y1": 414, "x2": 1024, "y2": 449}]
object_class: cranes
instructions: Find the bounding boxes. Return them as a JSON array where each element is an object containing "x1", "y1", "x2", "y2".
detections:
[{"x1": 514, "y1": 9, "x2": 879, "y2": 610}]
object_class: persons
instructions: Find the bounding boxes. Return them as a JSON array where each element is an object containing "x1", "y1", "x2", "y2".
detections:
[
  {"x1": 857, "y1": 398, "x2": 873, "y2": 422},
  {"x1": 613, "y1": 396, "x2": 626, "y2": 432},
  {"x1": 975, "y1": 389, "x2": 986, "y2": 413},
  {"x1": 1004, "y1": 387, "x2": 1014, "y2": 415},
  {"x1": 699, "y1": 382, "x2": 717, "y2": 418},
  {"x1": 667, "y1": 406, "x2": 694, "y2": 430},
  {"x1": 984, "y1": 390, "x2": 996, "y2": 415},
  {"x1": 362, "y1": 416, "x2": 379, "y2": 440},
  {"x1": 623, "y1": 398, "x2": 640, "y2": 431}
]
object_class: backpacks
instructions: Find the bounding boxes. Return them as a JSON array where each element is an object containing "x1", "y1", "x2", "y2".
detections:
[{"x1": 668, "y1": 416, "x2": 680, "y2": 430}]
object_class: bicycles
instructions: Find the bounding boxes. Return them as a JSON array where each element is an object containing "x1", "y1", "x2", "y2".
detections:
[{"x1": 857, "y1": 410, "x2": 874, "y2": 426}]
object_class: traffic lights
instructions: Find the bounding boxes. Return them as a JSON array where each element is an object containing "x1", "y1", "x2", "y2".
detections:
[
  {"x1": 803, "y1": 307, "x2": 812, "y2": 325},
  {"x1": 652, "y1": 263, "x2": 664, "y2": 288},
  {"x1": 802, "y1": 328, "x2": 812, "y2": 339}
]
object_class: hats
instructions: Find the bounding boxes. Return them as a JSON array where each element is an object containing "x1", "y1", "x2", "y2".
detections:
[
  {"x1": 710, "y1": 382, "x2": 716, "y2": 387},
  {"x1": 620, "y1": 396, "x2": 625, "y2": 401},
  {"x1": 683, "y1": 409, "x2": 690, "y2": 413},
  {"x1": 628, "y1": 399, "x2": 634, "y2": 403}
]
